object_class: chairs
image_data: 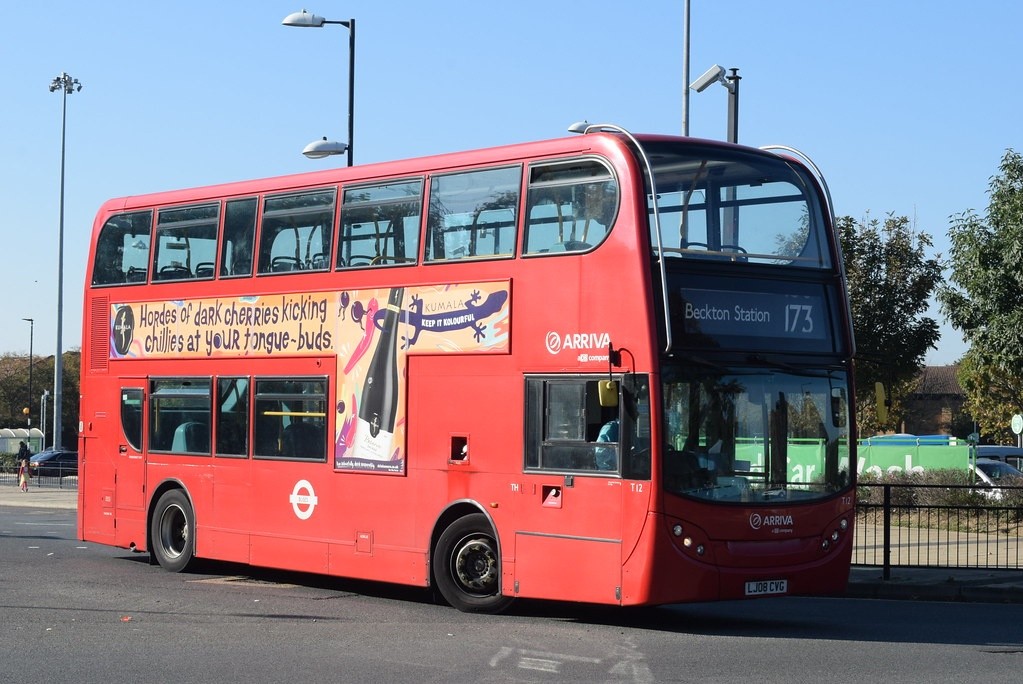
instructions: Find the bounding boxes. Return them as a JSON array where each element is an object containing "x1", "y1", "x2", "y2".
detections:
[
  {"x1": 169, "y1": 422, "x2": 324, "y2": 459},
  {"x1": 93, "y1": 253, "x2": 374, "y2": 284}
]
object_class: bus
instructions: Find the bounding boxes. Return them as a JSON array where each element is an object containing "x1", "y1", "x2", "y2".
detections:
[{"x1": 72, "y1": 123, "x2": 892, "y2": 616}]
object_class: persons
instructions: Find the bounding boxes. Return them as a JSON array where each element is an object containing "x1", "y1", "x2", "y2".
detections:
[
  {"x1": 17, "y1": 441, "x2": 33, "y2": 478},
  {"x1": 18, "y1": 459, "x2": 34, "y2": 493},
  {"x1": 594, "y1": 418, "x2": 671, "y2": 480}
]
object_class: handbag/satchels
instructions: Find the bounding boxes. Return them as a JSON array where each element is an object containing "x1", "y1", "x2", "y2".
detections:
[{"x1": 16, "y1": 455, "x2": 22, "y2": 463}]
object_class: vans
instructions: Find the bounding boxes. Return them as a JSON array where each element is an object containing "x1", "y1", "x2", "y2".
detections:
[
  {"x1": 967, "y1": 446, "x2": 1023, "y2": 470},
  {"x1": 862, "y1": 434, "x2": 965, "y2": 446}
]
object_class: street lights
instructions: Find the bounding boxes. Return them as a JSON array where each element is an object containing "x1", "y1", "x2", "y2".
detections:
[
  {"x1": 23, "y1": 407, "x2": 31, "y2": 453},
  {"x1": 282, "y1": 11, "x2": 357, "y2": 268},
  {"x1": 23, "y1": 317, "x2": 36, "y2": 458},
  {"x1": 47, "y1": 69, "x2": 83, "y2": 452}
]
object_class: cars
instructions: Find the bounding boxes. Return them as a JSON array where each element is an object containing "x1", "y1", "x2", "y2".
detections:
[
  {"x1": 968, "y1": 457, "x2": 1023, "y2": 497},
  {"x1": 28, "y1": 451, "x2": 78, "y2": 477}
]
